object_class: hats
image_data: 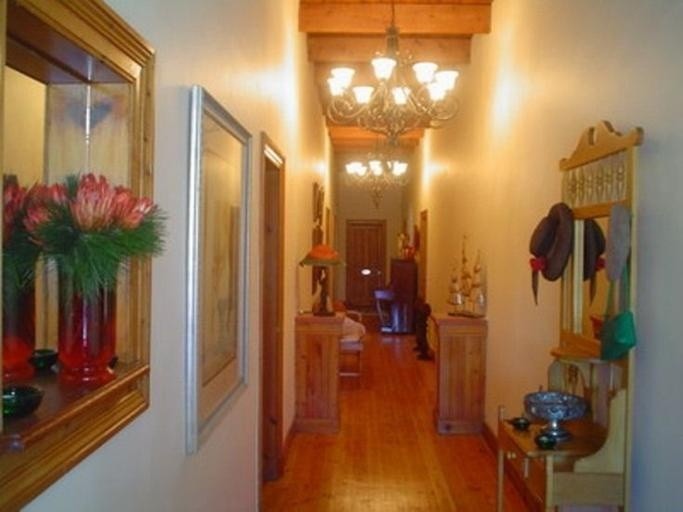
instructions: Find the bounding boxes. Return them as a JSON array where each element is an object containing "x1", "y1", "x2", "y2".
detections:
[{"x1": 530, "y1": 203, "x2": 574, "y2": 306}]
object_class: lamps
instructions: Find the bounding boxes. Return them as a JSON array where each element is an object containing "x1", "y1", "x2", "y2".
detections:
[
  {"x1": 320, "y1": 0, "x2": 463, "y2": 207},
  {"x1": 299, "y1": 241, "x2": 349, "y2": 317}
]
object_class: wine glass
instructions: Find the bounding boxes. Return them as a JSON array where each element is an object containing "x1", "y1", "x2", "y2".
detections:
[{"x1": 523, "y1": 391, "x2": 587, "y2": 442}]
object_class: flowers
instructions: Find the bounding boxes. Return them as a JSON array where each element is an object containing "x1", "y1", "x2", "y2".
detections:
[
  {"x1": 20, "y1": 173, "x2": 166, "y2": 298},
  {"x1": 4, "y1": 178, "x2": 41, "y2": 320}
]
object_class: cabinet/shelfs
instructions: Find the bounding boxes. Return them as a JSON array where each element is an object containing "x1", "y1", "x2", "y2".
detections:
[
  {"x1": 296, "y1": 311, "x2": 344, "y2": 434},
  {"x1": 428, "y1": 313, "x2": 488, "y2": 435}
]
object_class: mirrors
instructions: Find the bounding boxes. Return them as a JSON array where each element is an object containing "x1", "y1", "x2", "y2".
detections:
[
  {"x1": 548, "y1": 124, "x2": 647, "y2": 368},
  {"x1": 2, "y1": 1, "x2": 149, "y2": 509}
]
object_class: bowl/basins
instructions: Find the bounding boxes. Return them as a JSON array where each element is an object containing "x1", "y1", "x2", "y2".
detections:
[
  {"x1": 28, "y1": 348, "x2": 58, "y2": 371},
  {"x1": 511, "y1": 417, "x2": 530, "y2": 431},
  {"x1": 1, "y1": 385, "x2": 44, "y2": 417},
  {"x1": 535, "y1": 434, "x2": 556, "y2": 450}
]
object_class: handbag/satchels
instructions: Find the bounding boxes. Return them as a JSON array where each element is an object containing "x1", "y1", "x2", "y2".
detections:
[{"x1": 600, "y1": 263, "x2": 636, "y2": 361}]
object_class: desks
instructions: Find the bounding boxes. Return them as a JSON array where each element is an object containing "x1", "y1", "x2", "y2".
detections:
[{"x1": 495, "y1": 416, "x2": 630, "y2": 512}]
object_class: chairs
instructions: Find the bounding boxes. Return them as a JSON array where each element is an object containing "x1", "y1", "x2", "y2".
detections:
[{"x1": 371, "y1": 260, "x2": 418, "y2": 336}]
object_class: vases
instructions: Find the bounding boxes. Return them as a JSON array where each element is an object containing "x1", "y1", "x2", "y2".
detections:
[
  {"x1": 4, "y1": 292, "x2": 38, "y2": 394},
  {"x1": 55, "y1": 272, "x2": 119, "y2": 382}
]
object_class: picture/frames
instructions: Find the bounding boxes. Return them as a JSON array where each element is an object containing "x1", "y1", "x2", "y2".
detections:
[{"x1": 186, "y1": 84, "x2": 251, "y2": 452}]
